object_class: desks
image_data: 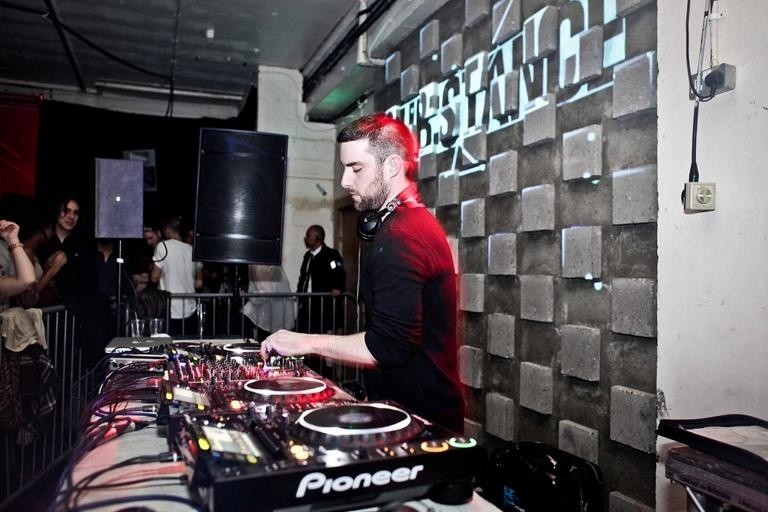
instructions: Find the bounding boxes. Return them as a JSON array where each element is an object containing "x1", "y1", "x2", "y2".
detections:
[{"x1": 55, "y1": 337, "x2": 505, "y2": 512}]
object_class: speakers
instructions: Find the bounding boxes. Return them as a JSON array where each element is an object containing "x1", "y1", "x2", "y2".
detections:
[
  {"x1": 192, "y1": 128, "x2": 288, "y2": 266},
  {"x1": 94, "y1": 157, "x2": 145, "y2": 240}
]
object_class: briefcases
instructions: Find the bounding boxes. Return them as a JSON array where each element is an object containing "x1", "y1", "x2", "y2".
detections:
[{"x1": 497, "y1": 441, "x2": 571, "y2": 511}]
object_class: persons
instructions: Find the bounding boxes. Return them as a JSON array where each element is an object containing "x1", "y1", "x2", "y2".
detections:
[
  {"x1": 260, "y1": 114, "x2": 466, "y2": 439},
  {"x1": 295, "y1": 224, "x2": 346, "y2": 385},
  {"x1": 2, "y1": 191, "x2": 204, "y2": 511}
]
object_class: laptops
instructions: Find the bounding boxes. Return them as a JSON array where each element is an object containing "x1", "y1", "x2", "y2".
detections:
[{"x1": 103, "y1": 336, "x2": 171, "y2": 353}]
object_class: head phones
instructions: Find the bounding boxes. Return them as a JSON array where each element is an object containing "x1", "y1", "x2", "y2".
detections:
[{"x1": 355, "y1": 181, "x2": 419, "y2": 241}]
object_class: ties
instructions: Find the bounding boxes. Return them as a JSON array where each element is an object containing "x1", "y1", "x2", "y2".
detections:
[{"x1": 304, "y1": 254, "x2": 312, "y2": 292}]
